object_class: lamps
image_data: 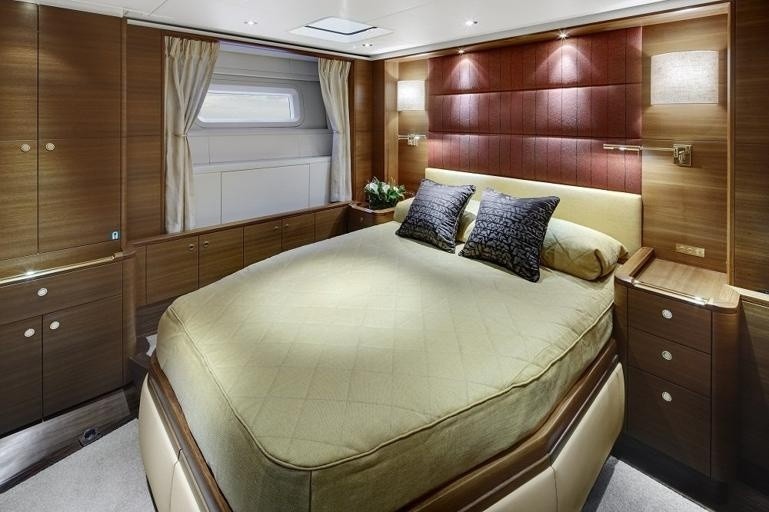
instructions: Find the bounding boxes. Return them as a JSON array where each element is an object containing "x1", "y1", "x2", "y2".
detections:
[
  {"x1": 397, "y1": 80, "x2": 425, "y2": 111},
  {"x1": 603, "y1": 144, "x2": 692, "y2": 167},
  {"x1": 651, "y1": 50, "x2": 719, "y2": 104},
  {"x1": 398, "y1": 128, "x2": 426, "y2": 147}
]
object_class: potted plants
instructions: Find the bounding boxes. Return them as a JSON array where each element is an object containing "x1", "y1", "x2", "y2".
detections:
[{"x1": 363, "y1": 174, "x2": 406, "y2": 210}]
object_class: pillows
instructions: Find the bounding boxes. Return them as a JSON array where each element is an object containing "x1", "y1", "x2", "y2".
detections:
[
  {"x1": 458, "y1": 187, "x2": 560, "y2": 282},
  {"x1": 395, "y1": 178, "x2": 475, "y2": 254},
  {"x1": 393, "y1": 197, "x2": 481, "y2": 244},
  {"x1": 540, "y1": 217, "x2": 631, "y2": 281}
]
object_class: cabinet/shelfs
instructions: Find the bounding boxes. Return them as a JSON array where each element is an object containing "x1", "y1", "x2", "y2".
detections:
[
  {"x1": 0, "y1": 0, "x2": 123, "y2": 438},
  {"x1": 129, "y1": 201, "x2": 358, "y2": 356}
]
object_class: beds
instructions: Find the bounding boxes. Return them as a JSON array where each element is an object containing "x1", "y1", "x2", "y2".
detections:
[{"x1": 137, "y1": 168, "x2": 643, "y2": 512}]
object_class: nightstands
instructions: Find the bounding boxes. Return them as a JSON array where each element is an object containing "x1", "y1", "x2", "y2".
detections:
[
  {"x1": 615, "y1": 246, "x2": 740, "y2": 484},
  {"x1": 348, "y1": 200, "x2": 395, "y2": 233}
]
